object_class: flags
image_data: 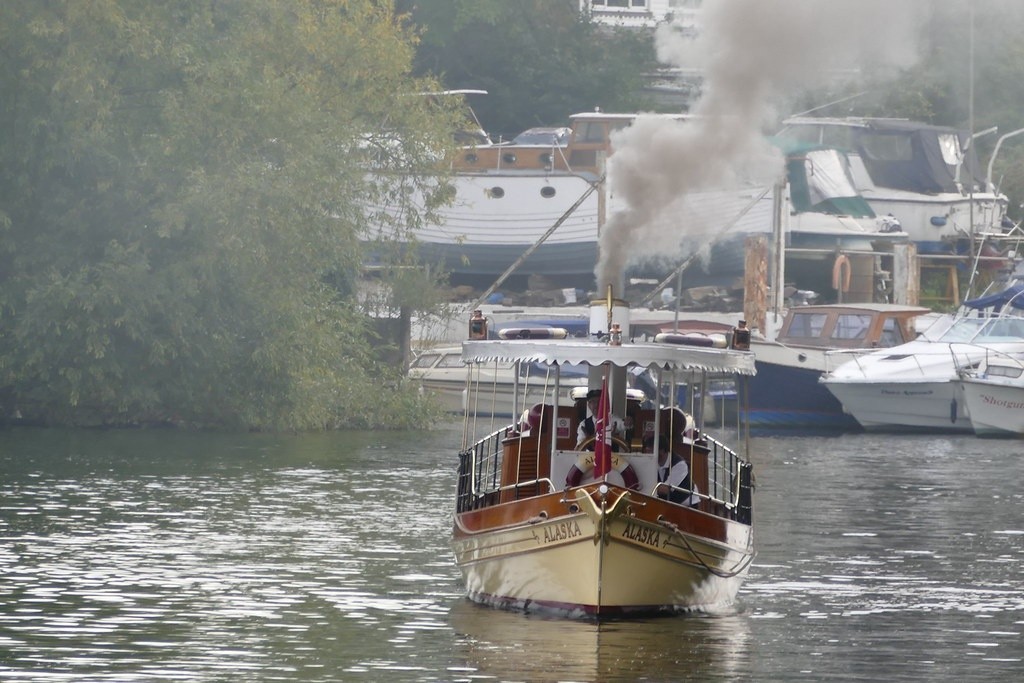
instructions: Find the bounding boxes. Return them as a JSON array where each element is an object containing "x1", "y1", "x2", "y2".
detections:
[{"x1": 592, "y1": 375, "x2": 612, "y2": 480}]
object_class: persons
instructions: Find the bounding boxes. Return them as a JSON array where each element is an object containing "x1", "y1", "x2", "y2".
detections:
[
  {"x1": 575, "y1": 389, "x2": 630, "y2": 454},
  {"x1": 644, "y1": 434, "x2": 701, "y2": 510}
]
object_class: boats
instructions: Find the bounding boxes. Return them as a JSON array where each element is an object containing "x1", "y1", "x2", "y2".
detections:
[
  {"x1": 449, "y1": 281, "x2": 755, "y2": 622},
  {"x1": 332, "y1": 89, "x2": 1024, "y2": 439}
]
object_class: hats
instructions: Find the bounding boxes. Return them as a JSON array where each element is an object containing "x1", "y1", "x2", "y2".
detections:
[{"x1": 586, "y1": 390, "x2": 601, "y2": 401}]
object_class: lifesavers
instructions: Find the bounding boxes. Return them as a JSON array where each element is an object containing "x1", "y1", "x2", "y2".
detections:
[
  {"x1": 565, "y1": 450, "x2": 639, "y2": 493},
  {"x1": 833, "y1": 254, "x2": 851, "y2": 293},
  {"x1": 498, "y1": 327, "x2": 567, "y2": 340},
  {"x1": 655, "y1": 333, "x2": 726, "y2": 348}
]
